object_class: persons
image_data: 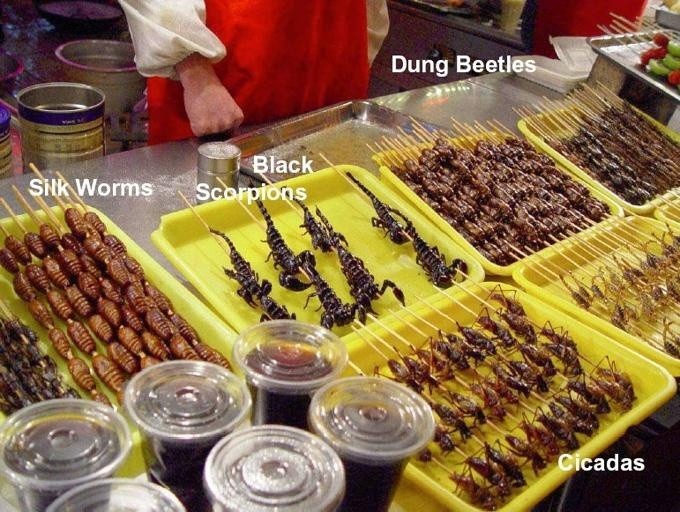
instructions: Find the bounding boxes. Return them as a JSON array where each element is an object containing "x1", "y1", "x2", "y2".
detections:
[
  {"x1": 522, "y1": 0, "x2": 649, "y2": 59},
  {"x1": 117, "y1": 0, "x2": 390, "y2": 146}
]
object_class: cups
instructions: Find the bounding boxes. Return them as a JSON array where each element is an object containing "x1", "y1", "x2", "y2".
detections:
[
  {"x1": 501, "y1": 0, "x2": 526, "y2": 32},
  {"x1": 0, "y1": 320, "x2": 436, "y2": 512}
]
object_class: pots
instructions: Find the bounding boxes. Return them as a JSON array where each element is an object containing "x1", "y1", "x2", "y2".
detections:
[{"x1": 34, "y1": 0, "x2": 147, "y2": 117}]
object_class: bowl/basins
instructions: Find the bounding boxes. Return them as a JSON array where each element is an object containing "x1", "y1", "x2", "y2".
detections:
[{"x1": 0, "y1": 47, "x2": 25, "y2": 97}]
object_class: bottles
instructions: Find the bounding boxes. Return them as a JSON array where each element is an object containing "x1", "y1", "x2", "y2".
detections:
[{"x1": 196, "y1": 141, "x2": 241, "y2": 205}]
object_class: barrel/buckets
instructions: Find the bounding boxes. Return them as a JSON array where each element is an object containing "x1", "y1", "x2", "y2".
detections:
[
  {"x1": 15, "y1": 82, "x2": 106, "y2": 176},
  {"x1": 1, "y1": 103, "x2": 14, "y2": 179},
  {"x1": 54, "y1": 37, "x2": 148, "y2": 157}
]
